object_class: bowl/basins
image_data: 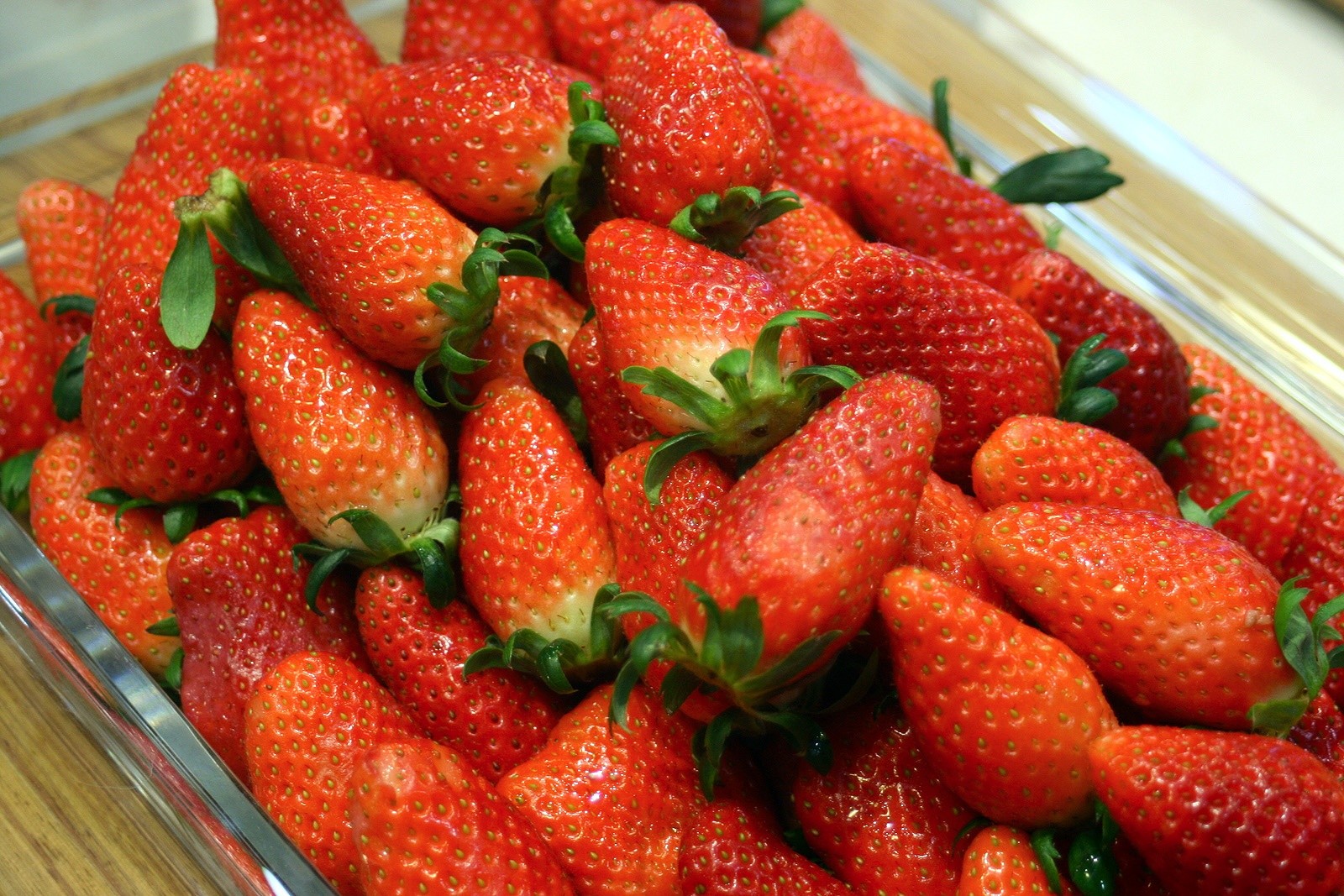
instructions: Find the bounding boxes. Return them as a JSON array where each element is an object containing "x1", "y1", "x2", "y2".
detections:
[{"x1": 3, "y1": 0, "x2": 1344, "y2": 896}]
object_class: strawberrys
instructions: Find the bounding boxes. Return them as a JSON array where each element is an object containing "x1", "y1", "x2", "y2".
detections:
[{"x1": 0, "y1": 0, "x2": 1344, "y2": 896}]
